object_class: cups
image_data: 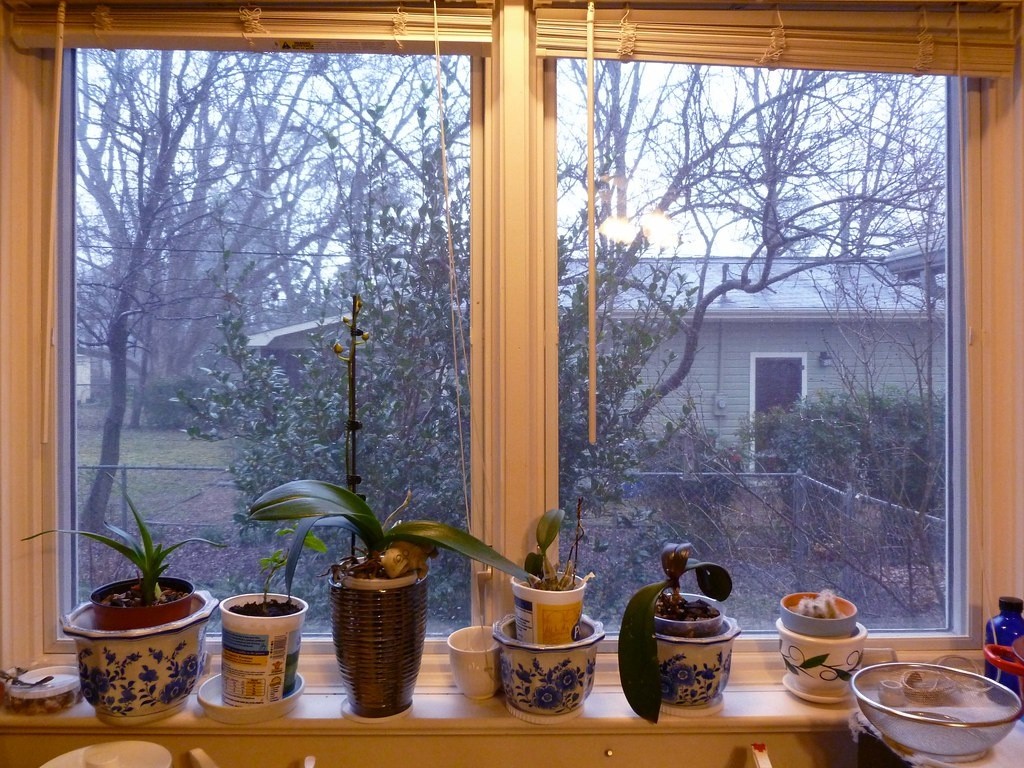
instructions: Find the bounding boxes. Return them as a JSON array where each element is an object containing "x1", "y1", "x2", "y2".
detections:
[
  {"x1": 447, "y1": 624, "x2": 500, "y2": 700},
  {"x1": 878, "y1": 680, "x2": 904, "y2": 706}
]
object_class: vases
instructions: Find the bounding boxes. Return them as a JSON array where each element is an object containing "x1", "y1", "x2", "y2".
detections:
[
  {"x1": 492, "y1": 611, "x2": 606, "y2": 715},
  {"x1": 655, "y1": 614, "x2": 742, "y2": 718},
  {"x1": 776, "y1": 618, "x2": 868, "y2": 694},
  {"x1": 447, "y1": 626, "x2": 504, "y2": 701},
  {"x1": 59, "y1": 589, "x2": 219, "y2": 727}
]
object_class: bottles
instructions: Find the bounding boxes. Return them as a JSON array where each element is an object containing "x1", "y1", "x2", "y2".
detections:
[{"x1": 984, "y1": 596, "x2": 1024, "y2": 708}]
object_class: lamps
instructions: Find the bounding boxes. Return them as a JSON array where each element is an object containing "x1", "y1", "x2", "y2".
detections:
[{"x1": 599, "y1": 167, "x2": 686, "y2": 248}]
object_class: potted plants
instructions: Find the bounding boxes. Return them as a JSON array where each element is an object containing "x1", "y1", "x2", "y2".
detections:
[
  {"x1": 247, "y1": 479, "x2": 533, "y2": 716},
  {"x1": 780, "y1": 591, "x2": 858, "y2": 639},
  {"x1": 617, "y1": 542, "x2": 732, "y2": 726},
  {"x1": 21, "y1": 471, "x2": 227, "y2": 631},
  {"x1": 511, "y1": 497, "x2": 596, "y2": 646},
  {"x1": 218, "y1": 519, "x2": 327, "y2": 710}
]
